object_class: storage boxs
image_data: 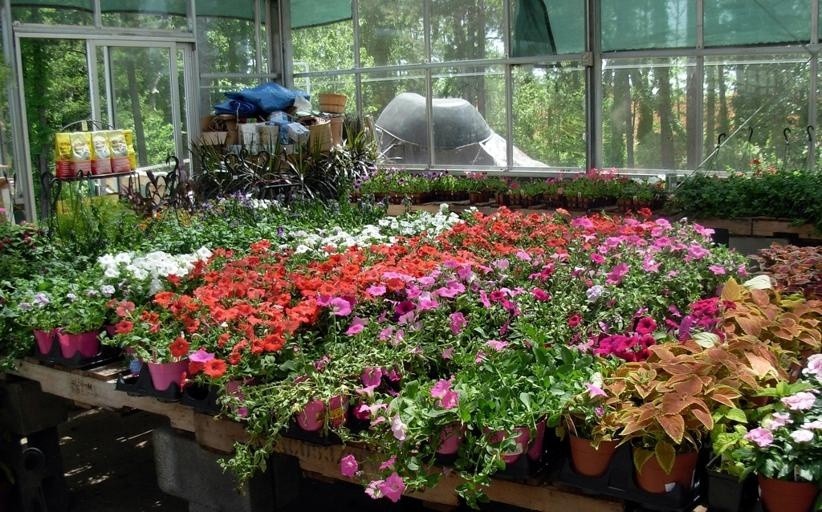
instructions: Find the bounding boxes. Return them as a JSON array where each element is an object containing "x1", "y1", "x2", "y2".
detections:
[{"x1": 199, "y1": 112, "x2": 332, "y2": 160}]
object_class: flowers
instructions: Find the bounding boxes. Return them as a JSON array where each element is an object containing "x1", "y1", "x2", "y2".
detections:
[{"x1": 1, "y1": 171, "x2": 822, "y2": 511}]
